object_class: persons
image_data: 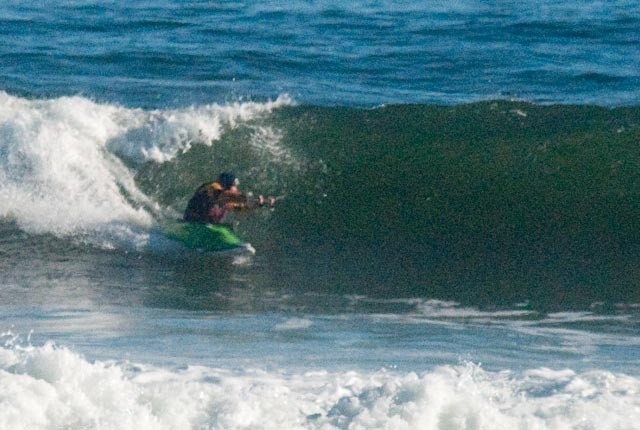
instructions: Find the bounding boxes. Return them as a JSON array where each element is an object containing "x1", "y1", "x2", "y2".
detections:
[{"x1": 183, "y1": 172, "x2": 276, "y2": 223}]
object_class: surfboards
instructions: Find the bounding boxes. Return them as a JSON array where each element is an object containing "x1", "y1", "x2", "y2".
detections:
[{"x1": 161, "y1": 223, "x2": 256, "y2": 255}]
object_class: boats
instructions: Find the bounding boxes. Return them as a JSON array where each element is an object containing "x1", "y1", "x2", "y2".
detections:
[{"x1": 156, "y1": 217, "x2": 257, "y2": 258}]
